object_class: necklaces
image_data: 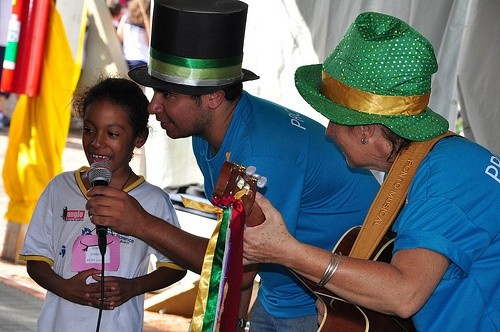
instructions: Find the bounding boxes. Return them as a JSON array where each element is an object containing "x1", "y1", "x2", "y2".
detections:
[{"x1": 116, "y1": 168, "x2": 132, "y2": 193}]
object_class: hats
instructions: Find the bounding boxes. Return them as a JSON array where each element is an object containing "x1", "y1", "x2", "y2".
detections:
[
  {"x1": 128, "y1": 0, "x2": 260, "y2": 95},
  {"x1": 294, "y1": 12, "x2": 449, "y2": 142}
]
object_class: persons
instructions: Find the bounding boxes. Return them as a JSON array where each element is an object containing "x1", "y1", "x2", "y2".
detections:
[
  {"x1": 242, "y1": 12, "x2": 500, "y2": 332},
  {"x1": 1, "y1": 1, "x2": 154, "y2": 266},
  {"x1": 18, "y1": 77, "x2": 189, "y2": 332},
  {"x1": 84, "y1": 0, "x2": 383, "y2": 332}
]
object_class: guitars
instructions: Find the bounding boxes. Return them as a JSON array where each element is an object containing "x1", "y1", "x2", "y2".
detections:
[{"x1": 212, "y1": 161, "x2": 418, "y2": 332}]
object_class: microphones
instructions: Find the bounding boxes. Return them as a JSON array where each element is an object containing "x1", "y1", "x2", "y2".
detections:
[{"x1": 87, "y1": 162, "x2": 113, "y2": 256}]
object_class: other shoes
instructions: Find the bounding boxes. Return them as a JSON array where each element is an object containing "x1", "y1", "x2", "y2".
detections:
[
  {"x1": 0, "y1": 112, "x2": 10, "y2": 132},
  {"x1": 70, "y1": 115, "x2": 82, "y2": 129}
]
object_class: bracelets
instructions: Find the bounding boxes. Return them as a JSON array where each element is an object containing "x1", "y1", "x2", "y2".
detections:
[{"x1": 315, "y1": 252, "x2": 342, "y2": 288}]
